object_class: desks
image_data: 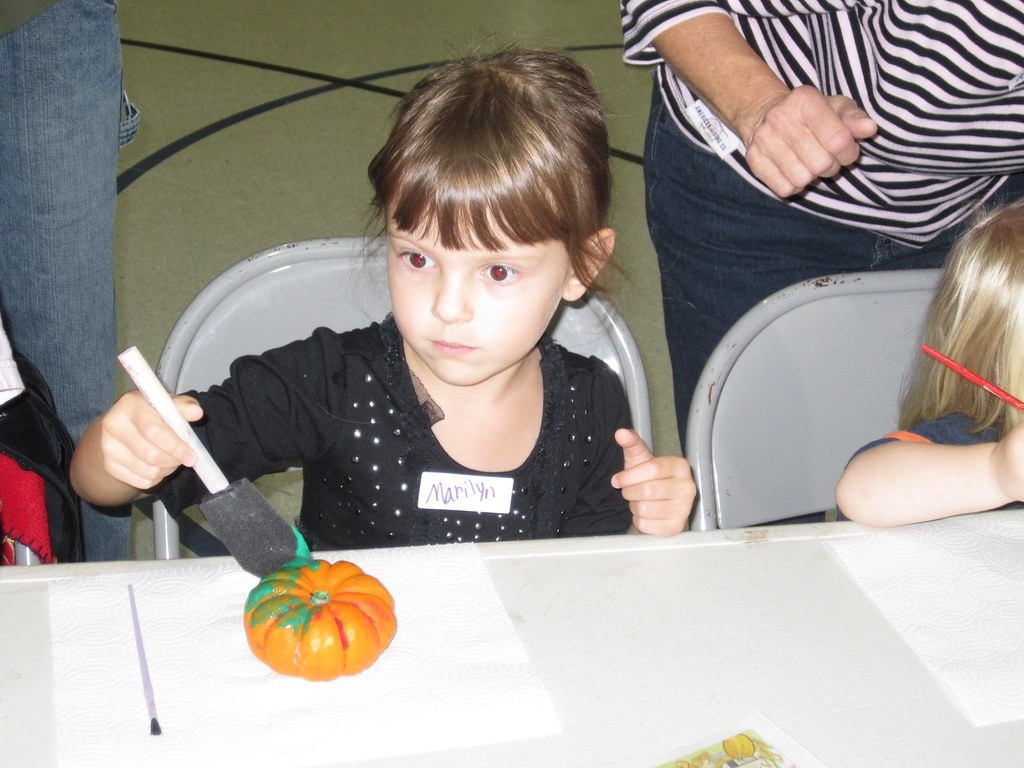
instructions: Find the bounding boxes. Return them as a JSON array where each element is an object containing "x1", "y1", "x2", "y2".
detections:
[{"x1": 1, "y1": 509, "x2": 1024, "y2": 768}]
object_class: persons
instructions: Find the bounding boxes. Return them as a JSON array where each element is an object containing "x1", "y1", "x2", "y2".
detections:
[
  {"x1": 835, "y1": 203, "x2": 1024, "y2": 529},
  {"x1": 619, "y1": 0, "x2": 1024, "y2": 527},
  {"x1": 70, "y1": 40, "x2": 698, "y2": 550},
  {"x1": 0, "y1": 0, "x2": 140, "y2": 564}
]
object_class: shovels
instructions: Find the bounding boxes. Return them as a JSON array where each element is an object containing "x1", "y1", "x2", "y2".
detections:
[{"x1": 118, "y1": 345, "x2": 312, "y2": 577}]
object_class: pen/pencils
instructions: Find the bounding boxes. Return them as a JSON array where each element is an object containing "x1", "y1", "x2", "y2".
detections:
[{"x1": 920, "y1": 344, "x2": 1024, "y2": 412}]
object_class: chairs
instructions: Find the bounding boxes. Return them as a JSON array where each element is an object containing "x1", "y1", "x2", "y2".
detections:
[
  {"x1": 686, "y1": 271, "x2": 950, "y2": 531},
  {"x1": 153, "y1": 238, "x2": 653, "y2": 562}
]
